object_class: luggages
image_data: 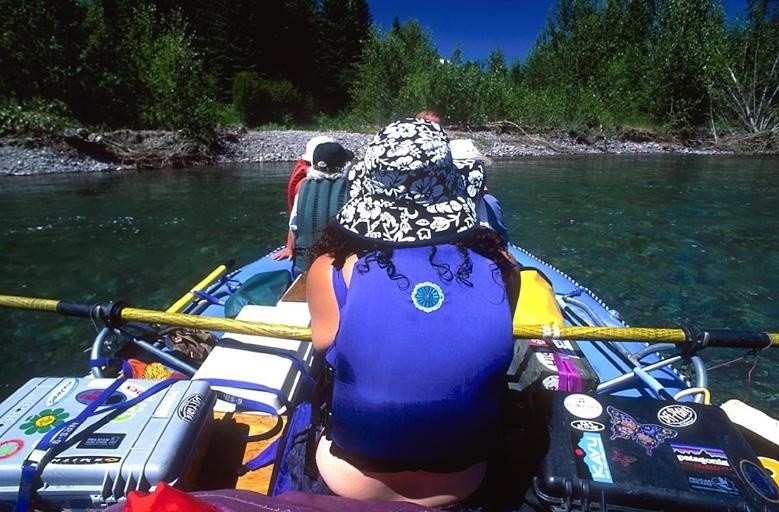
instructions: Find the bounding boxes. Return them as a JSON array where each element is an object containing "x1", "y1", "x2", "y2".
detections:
[
  {"x1": 523, "y1": 385, "x2": 778, "y2": 511},
  {"x1": 0, "y1": 376, "x2": 219, "y2": 506}
]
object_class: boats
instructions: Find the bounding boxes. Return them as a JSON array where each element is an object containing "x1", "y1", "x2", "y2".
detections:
[{"x1": 0, "y1": 228, "x2": 777, "y2": 512}]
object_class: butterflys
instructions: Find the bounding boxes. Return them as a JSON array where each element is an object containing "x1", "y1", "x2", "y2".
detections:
[{"x1": 606, "y1": 405, "x2": 678, "y2": 468}]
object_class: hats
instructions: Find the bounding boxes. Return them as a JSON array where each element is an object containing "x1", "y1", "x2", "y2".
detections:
[
  {"x1": 332, "y1": 117, "x2": 486, "y2": 245},
  {"x1": 448, "y1": 139, "x2": 491, "y2": 166},
  {"x1": 301, "y1": 136, "x2": 355, "y2": 168}
]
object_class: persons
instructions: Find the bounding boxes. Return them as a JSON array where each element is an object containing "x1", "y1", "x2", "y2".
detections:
[
  {"x1": 447, "y1": 133, "x2": 510, "y2": 247},
  {"x1": 285, "y1": 137, "x2": 334, "y2": 215},
  {"x1": 304, "y1": 118, "x2": 524, "y2": 509},
  {"x1": 272, "y1": 142, "x2": 358, "y2": 278},
  {"x1": 413, "y1": 108, "x2": 442, "y2": 128}
]
